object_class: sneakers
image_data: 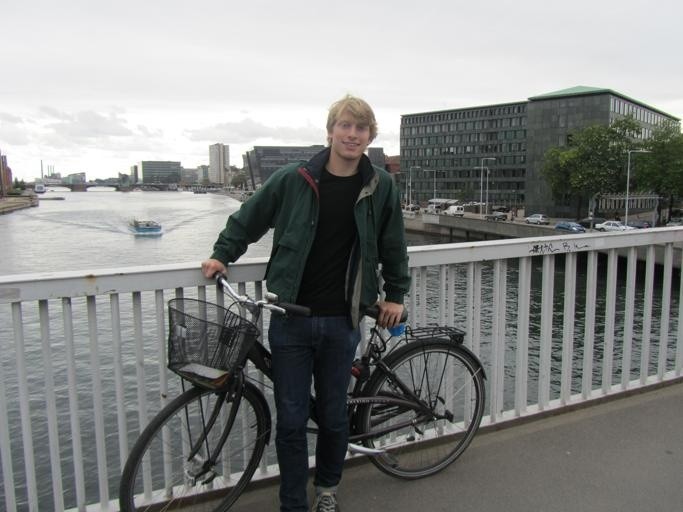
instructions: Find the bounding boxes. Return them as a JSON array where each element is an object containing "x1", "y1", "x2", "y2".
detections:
[{"x1": 312, "y1": 492, "x2": 337, "y2": 512}]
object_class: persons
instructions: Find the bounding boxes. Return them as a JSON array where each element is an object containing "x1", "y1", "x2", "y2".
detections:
[{"x1": 201, "y1": 96, "x2": 412, "y2": 511}]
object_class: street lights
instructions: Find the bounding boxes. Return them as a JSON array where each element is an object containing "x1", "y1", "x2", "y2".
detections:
[
  {"x1": 409, "y1": 165, "x2": 421, "y2": 212},
  {"x1": 424, "y1": 170, "x2": 436, "y2": 210},
  {"x1": 625, "y1": 150, "x2": 651, "y2": 226},
  {"x1": 474, "y1": 166, "x2": 489, "y2": 216},
  {"x1": 480, "y1": 157, "x2": 496, "y2": 219},
  {"x1": 394, "y1": 172, "x2": 407, "y2": 210}
]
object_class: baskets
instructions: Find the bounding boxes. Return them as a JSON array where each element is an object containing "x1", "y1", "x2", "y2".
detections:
[{"x1": 167, "y1": 298, "x2": 260, "y2": 392}]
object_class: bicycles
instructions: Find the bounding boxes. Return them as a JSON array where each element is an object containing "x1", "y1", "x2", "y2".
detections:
[{"x1": 117, "y1": 267, "x2": 488, "y2": 511}]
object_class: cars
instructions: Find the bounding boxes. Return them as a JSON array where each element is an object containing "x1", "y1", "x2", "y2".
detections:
[
  {"x1": 463, "y1": 201, "x2": 485, "y2": 208},
  {"x1": 524, "y1": 213, "x2": 551, "y2": 226},
  {"x1": 408, "y1": 204, "x2": 421, "y2": 212},
  {"x1": 555, "y1": 221, "x2": 585, "y2": 234},
  {"x1": 578, "y1": 217, "x2": 608, "y2": 229},
  {"x1": 492, "y1": 205, "x2": 509, "y2": 213},
  {"x1": 424, "y1": 205, "x2": 441, "y2": 214},
  {"x1": 400, "y1": 202, "x2": 407, "y2": 210},
  {"x1": 593, "y1": 221, "x2": 636, "y2": 231},
  {"x1": 637, "y1": 220, "x2": 651, "y2": 228},
  {"x1": 665, "y1": 220, "x2": 683, "y2": 226},
  {"x1": 484, "y1": 211, "x2": 507, "y2": 222}
]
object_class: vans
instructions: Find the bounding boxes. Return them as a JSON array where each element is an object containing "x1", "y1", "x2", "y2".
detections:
[{"x1": 443, "y1": 205, "x2": 465, "y2": 218}]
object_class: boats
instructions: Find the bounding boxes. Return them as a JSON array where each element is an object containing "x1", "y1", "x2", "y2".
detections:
[
  {"x1": 125, "y1": 219, "x2": 163, "y2": 239},
  {"x1": 193, "y1": 186, "x2": 207, "y2": 194}
]
